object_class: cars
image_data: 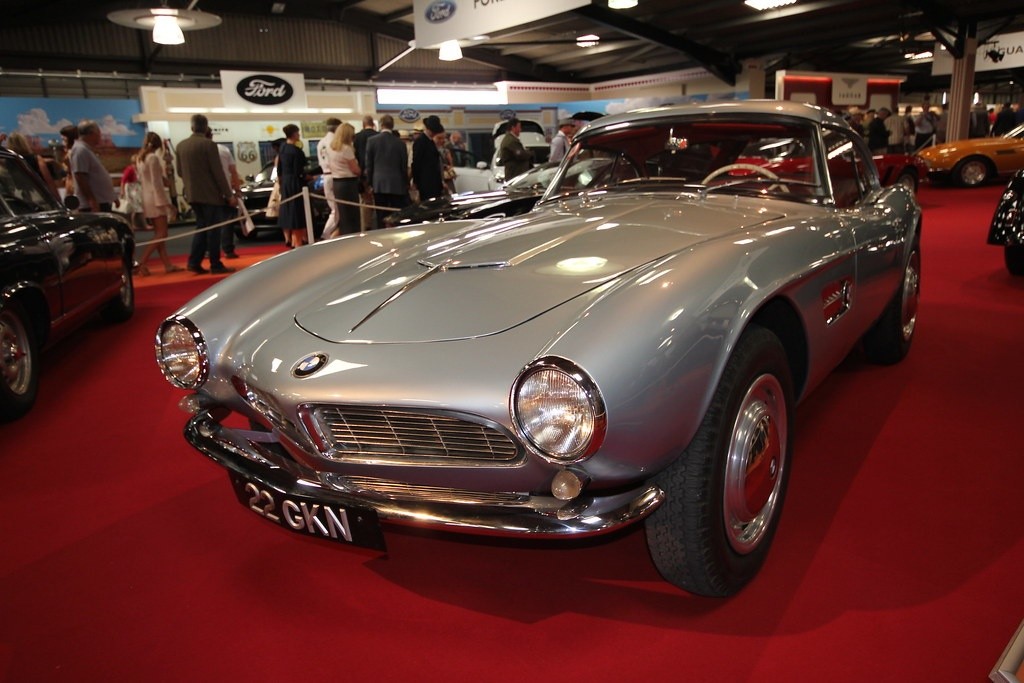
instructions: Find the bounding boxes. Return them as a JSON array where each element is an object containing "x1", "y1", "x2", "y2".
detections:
[
  {"x1": 383, "y1": 158, "x2": 672, "y2": 229},
  {"x1": 230, "y1": 148, "x2": 501, "y2": 241},
  {"x1": 987, "y1": 166, "x2": 1024, "y2": 277},
  {"x1": 14, "y1": 156, "x2": 125, "y2": 212},
  {"x1": 917, "y1": 122, "x2": 1024, "y2": 187},
  {"x1": 489, "y1": 118, "x2": 551, "y2": 190}
]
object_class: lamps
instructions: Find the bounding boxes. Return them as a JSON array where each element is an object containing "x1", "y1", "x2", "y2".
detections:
[
  {"x1": 107, "y1": 0, "x2": 222, "y2": 45},
  {"x1": 408, "y1": 34, "x2": 490, "y2": 61},
  {"x1": 477, "y1": 34, "x2": 601, "y2": 48}
]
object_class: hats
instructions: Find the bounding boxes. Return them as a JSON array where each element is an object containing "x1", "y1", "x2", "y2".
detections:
[
  {"x1": 423, "y1": 116, "x2": 444, "y2": 132},
  {"x1": 559, "y1": 119, "x2": 575, "y2": 127}
]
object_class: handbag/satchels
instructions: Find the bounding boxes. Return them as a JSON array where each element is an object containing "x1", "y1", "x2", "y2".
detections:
[
  {"x1": 238, "y1": 198, "x2": 254, "y2": 236},
  {"x1": 112, "y1": 199, "x2": 132, "y2": 215}
]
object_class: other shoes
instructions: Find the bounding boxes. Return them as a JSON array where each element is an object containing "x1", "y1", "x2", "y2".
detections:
[
  {"x1": 187, "y1": 263, "x2": 209, "y2": 273},
  {"x1": 146, "y1": 224, "x2": 154, "y2": 229},
  {"x1": 166, "y1": 265, "x2": 185, "y2": 272},
  {"x1": 212, "y1": 266, "x2": 235, "y2": 272}
]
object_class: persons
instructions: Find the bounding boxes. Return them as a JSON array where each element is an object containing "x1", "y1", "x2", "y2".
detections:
[
  {"x1": 827, "y1": 102, "x2": 1024, "y2": 154},
  {"x1": 136, "y1": 130, "x2": 185, "y2": 277},
  {"x1": 175, "y1": 113, "x2": 241, "y2": 275},
  {"x1": 501, "y1": 119, "x2": 535, "y2": 182},
  {"x1": 121, "y1": 153, "x2": 155, "y2": 232},
  {"x1": 265, "y1": 114, "x2": 468, "y2": 249},
  {"x1": 548, "y1": 115, "x2": 576, "y2": 163},
  {"x1": 5, "y1": 119, "x2": 118, "y2": 215}
]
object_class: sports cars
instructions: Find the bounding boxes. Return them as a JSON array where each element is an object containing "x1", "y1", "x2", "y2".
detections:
[
  {"x1": 154, "y1": 99, "x2": 922, "y2": 597},
  {"x1": 727, "y1": 110, "x2": 926, "y2": 208},
  {"x1": 0, "y1": 146, "x2": 136, "y2": 419}
]
